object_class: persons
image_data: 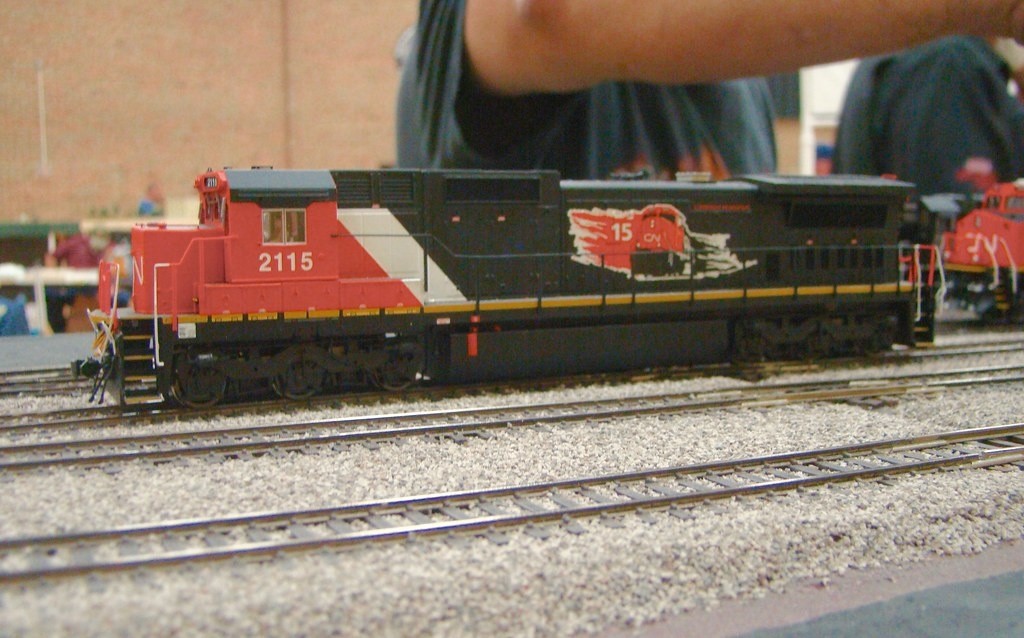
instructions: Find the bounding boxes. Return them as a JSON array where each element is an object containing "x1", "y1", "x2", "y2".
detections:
[
  {"x1": 46, "y1": 227, "x2": 119, "y2": 332},
  {"x1": 833, "y1": 33, "x2": 1024, "y2": 185},
  {"x1": 394, "y1": 0, "x2": 1024, "y2": 182}
]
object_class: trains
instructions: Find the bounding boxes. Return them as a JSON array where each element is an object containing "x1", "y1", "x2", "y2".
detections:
[
  {"x1": 69, "y1": 162, "x2": 940, "y2": 410},
  {"x1": 933, "y1": 176, "x2": 1024, "y2": 330}
]
